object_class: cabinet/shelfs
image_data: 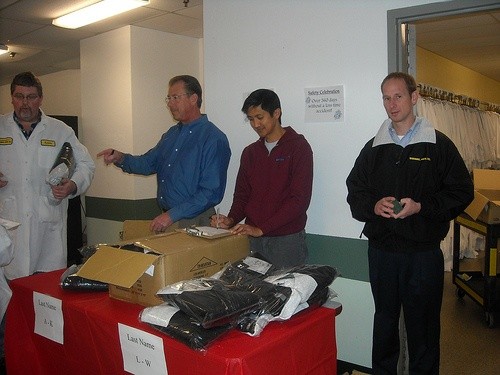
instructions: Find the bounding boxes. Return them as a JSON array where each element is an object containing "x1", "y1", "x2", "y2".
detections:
[{"x1": 452, "y1": 214, "x2": 500, "y2": 328}]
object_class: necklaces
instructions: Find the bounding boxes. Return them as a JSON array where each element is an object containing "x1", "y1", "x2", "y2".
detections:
[{"x1": 266, "y1": 130, "x2": 285, "y2": 143}]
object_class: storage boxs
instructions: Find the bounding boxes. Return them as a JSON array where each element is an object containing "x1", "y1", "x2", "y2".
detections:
[
  {"x1": 464, "y1": 168, "x2": 500, "y2": 223},
  {"x1": 76, "y1": 219, "x2": 250, "y2": 306}
]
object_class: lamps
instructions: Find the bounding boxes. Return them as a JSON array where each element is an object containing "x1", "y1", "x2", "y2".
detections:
[{"x1": 52, "y1": 0, "x2": 150, "y2": 29}]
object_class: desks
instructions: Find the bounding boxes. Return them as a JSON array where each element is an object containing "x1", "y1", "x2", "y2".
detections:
[{"x1": 1, "y1": 268, "x2": 342, "y2": 375}]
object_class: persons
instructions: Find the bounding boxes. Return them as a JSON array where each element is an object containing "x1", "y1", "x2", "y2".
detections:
[
  {"x1": 346, "y1": 73, "x2": 474, "y2": 375},
  {"x1": 97, "y1": 75, "x2": 232, "y2": 233},
  {"x1": 210, "y1": 89, "x2": 313, "y2": 272},
  {"x1": 0, "y1": 225, "x2": 22, "y2": 375},
  {"x1": 0, "y1": 72, "x2": 96, "y2": 282}
]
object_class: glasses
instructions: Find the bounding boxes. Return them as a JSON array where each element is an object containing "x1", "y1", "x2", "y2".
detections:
[{"x1": 164, "y1": 92, "x2": 193, "y2": 102}]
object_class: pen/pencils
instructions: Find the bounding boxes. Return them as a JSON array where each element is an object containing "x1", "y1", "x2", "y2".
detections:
[
  {"x1": 107, "y1": 149, "x2": 114, "y2": 166},
  {"x1": 216, "y1": 207, "x2": 220, "y2": 229}
]
